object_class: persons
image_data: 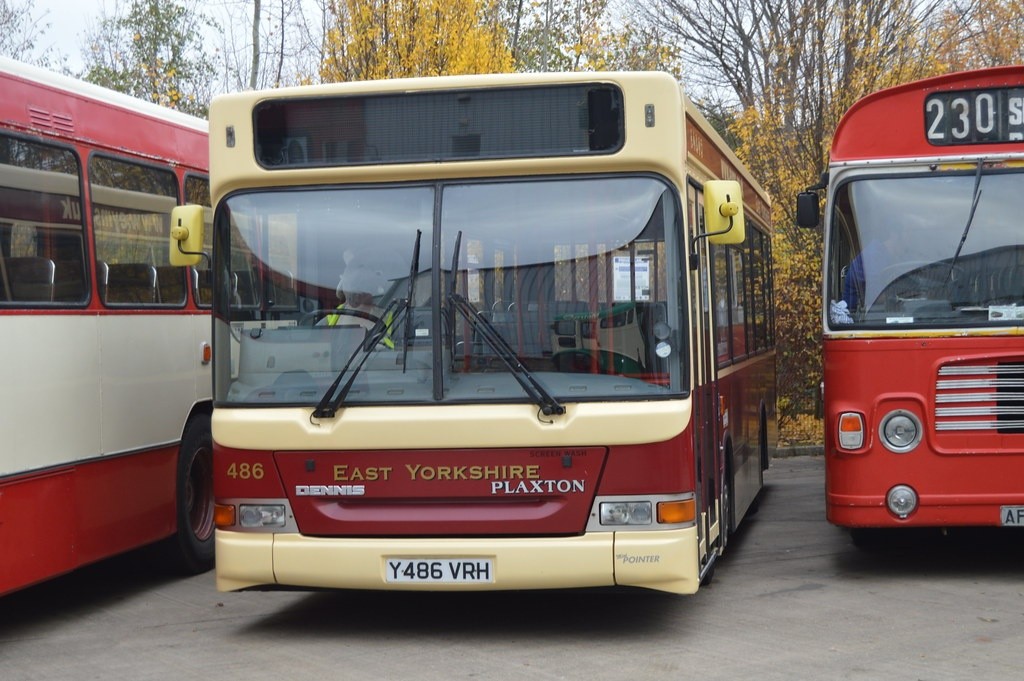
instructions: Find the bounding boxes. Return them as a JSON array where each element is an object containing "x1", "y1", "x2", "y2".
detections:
[
  {"x1": 318, "y1": 284, "x2": 396, "y2": 353},
  {"x1": 843, "y1": 217, "x2": 907, "y2": 322}
]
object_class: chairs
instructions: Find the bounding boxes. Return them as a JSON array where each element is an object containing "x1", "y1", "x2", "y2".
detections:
[
  {"x1": 392, "y1": 300, "x2": 670, "y2": 376},
  {"x1": 967, "y1": 264, "x2": 1024, "y2": 304},
  {"x1": 1, "y1": 257, "x2": 295, "y2": 306}
]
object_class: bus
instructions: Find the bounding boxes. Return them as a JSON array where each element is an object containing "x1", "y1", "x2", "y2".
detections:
[
  {"x1": 0, "y1": 54, "x2": 314, "y2": 599},
  {"x1": 165, "y1": 72, "x2": 775, "y2": 602},
  {"x1": 796, "y1": 62, "x2": 1024, "y2": 549}
]
